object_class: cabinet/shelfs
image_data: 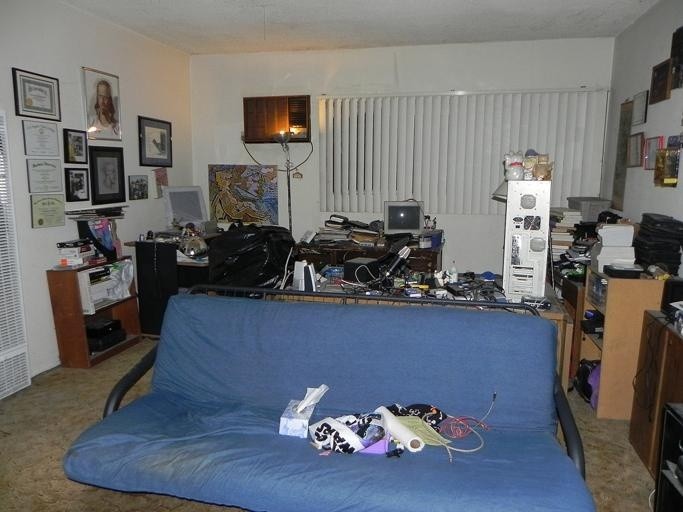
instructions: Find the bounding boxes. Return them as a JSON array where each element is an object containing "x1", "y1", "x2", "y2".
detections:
[
  {"x1": 624, "y1": 309, "x2": 682, "y2": 482},
  {"x1": 645, "y1": 404, "x2": 682, "y2": 512},
  {"x1": 41, "y1": 253, "x2": 145, "y2": 367},
  {"x1": 575, "y1": 265, "x2": 668, "y2": 423},
  {"x1": 552, "y1": 271, "x2": 583, "y2": 385},
  {"x1": 296, "y1": 229, "x2": 445, "y2": 274}
]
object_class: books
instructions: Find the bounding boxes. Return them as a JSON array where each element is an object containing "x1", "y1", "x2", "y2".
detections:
[
  {"x1": 298, "y1": 214, "x2": 385, "y2": 253},
  {"x1": 52, "y1": 236, "x2": 119, "y2": 312},
  {"x1": 547, "y1": 203, "x2": 583, "y2": 264}
]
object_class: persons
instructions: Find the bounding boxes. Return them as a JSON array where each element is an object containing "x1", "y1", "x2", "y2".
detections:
[
  {"x1": 99, "y1": 160, "x2": 115, "y2": 188},
  {"x1": 89, "y1": 78, "x2": 116, "y2": 138}
]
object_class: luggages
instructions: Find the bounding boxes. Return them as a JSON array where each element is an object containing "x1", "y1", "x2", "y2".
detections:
[
  {"x1": 574, "y1": 358, "x2": 601, "y2": 404},
  {"x1": 207, "y1": 223, "x2": 301, "y2": 286}
]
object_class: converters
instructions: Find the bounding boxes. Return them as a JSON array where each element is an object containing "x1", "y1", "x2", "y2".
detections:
[{"x1": 446, "y1": 284, "x2": 464, "y2": 296}]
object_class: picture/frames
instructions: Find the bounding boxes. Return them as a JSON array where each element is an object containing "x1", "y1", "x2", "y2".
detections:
[
  {"x1": 81, "y1": 65, "x2": 123, "y2": 141},
  {"x1": 623, "y1": 53, "x2": 683, "y2": 188},
  {"x1": 63, "y1": 166, "x2": 89, "y2": 202},
  {"x1": 11, "y1": 66, "x2": 66, "y2": 230},
  {"x1": 61, "y1": 127, "x2": 88, "y2": 165},
  {"x1": 136, "y1": 113, "x2": 172, "y2": 169},
  {"x1": 88, "y1": 144, "x2": 127, "y2": 205}
]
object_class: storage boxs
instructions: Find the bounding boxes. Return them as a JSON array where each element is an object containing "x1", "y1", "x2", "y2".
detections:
[{"x1": 566, "y1": 196, "x2": 612, "y2": 224}]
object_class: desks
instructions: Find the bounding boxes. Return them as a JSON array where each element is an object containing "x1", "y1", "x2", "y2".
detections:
[
  {"x1": 266, "y1": 270, "x2": 573, "y2": 448},
  {"x1": 130, "y1": 223, "x2": 229, "y2": 336}
]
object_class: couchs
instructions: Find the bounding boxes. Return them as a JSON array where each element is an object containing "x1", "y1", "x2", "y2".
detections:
[{"x1": 56, "y1": 282, "x2": 598, "y2": 512}]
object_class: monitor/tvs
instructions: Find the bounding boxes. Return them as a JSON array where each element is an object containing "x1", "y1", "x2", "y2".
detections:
[
  {"x1": 163, "y1": 186, "x2": 207, "y2": 227},
  {"x1": 383, "y1": 201, "x2": 424, "y2": 235}
]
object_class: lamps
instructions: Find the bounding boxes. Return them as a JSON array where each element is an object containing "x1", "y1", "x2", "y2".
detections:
[{"x1": 272, "y1": 130, "x2": 297, "y2": 238}]
